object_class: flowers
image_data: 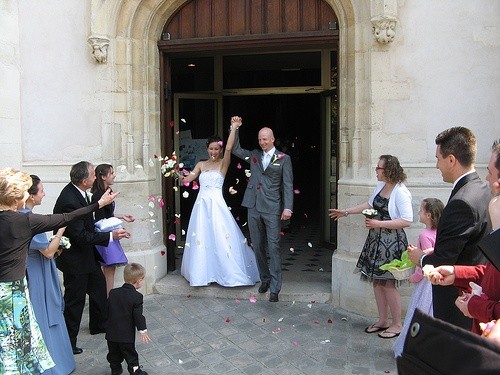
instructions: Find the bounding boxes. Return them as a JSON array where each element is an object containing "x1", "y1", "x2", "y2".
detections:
[
  {"x1": 48, "y1": 234, "x2": 71, "y2": 259},
  {"x1": 160, "y1": 158, "x2": 179, "y2": 179},
  {"x1": 361, "y1": 207, "x2": 380, "y2": 232},
  {"x1": 271, "y1": 153, "x2": 286, "y2": 164}
]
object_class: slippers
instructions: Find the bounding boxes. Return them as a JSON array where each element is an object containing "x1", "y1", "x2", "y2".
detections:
[
  {"x1": 365, "y1": 323, "x2": 389, "y2": 333},
  {"x1": 378, "y1": 330, "x2": 400, "y2": 338}
]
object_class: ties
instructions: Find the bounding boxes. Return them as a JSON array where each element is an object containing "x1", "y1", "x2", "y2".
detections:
[
  {"x1": 85, "y1": 192, "x2": 93, "y2": 218},
  {"x1": 263, "y1": 154, "x2": 268, "y2": 171}
]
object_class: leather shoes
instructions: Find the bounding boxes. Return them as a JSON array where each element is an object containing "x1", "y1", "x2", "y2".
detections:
[
  {"x1": 270, "y1": 293, "x2": 279, "y2": 302},
  {"x1": 259, "y1": 282, "x2": 270, "y2": 293}
]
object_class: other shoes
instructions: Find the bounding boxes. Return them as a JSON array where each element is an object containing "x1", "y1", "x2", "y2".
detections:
[
  {"x1": 90, "y1": 329, "x2": 107, "y2": 335},
  {"x1": 129, "y1": 365, "x2": 148, "y2": 375},
  {"x1": 73, "y1": 347, "x2": 83, "y2": 354},
  {"x1": 111, "y1": 369, "x2": 123, "y2": 375}
]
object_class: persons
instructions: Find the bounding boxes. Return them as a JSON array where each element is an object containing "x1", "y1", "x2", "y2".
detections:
[
  {"x1": 91, "y1": 164, "x2": 135, "y2": 299},
  {"x1": 408, "y1": 126, "x2": 492, "y2": 332},
  {"x1": 428, "y1": 138, "x2": 500, "y2": 343},
  {"x1": 0, "y1": 167, "x2": 121, "y2": 375},
  {"x1": 390, "y1": 198, "x2": 444, "y2": 358},
  {"x1": 175, "y1": 117, "x2": 260, "y2": 287},
  {"x1": 328, "y1": 154, "x2": 414, "y2": 338},
  {"x1": 229, "y1": 116, "x2": 293, "y2": 302},
  {"x1": 54, "y1": 161, "x2": 107, "y2": 354},
  {"x1": 101, "y1": 263, "x2": 151, "y2": 375},
  {"x1": 16, "y1": 174, "x2": 76, "y2": 375}
]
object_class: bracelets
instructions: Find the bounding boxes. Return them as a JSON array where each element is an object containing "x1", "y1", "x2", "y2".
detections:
[
  {"x1": 345, "y1": 208, "x2": 348, "y2": 217},
  {"x1": 55, "y1": 236, "x2": 61, "y2": 240},
  {"x1": 101, "y1": 199, "x2": 106, "y2": 206}
]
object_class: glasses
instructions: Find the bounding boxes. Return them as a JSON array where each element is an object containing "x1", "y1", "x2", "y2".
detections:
[{"x1": 375, "y1": 167, "x2": 383, "y2": 171}]
